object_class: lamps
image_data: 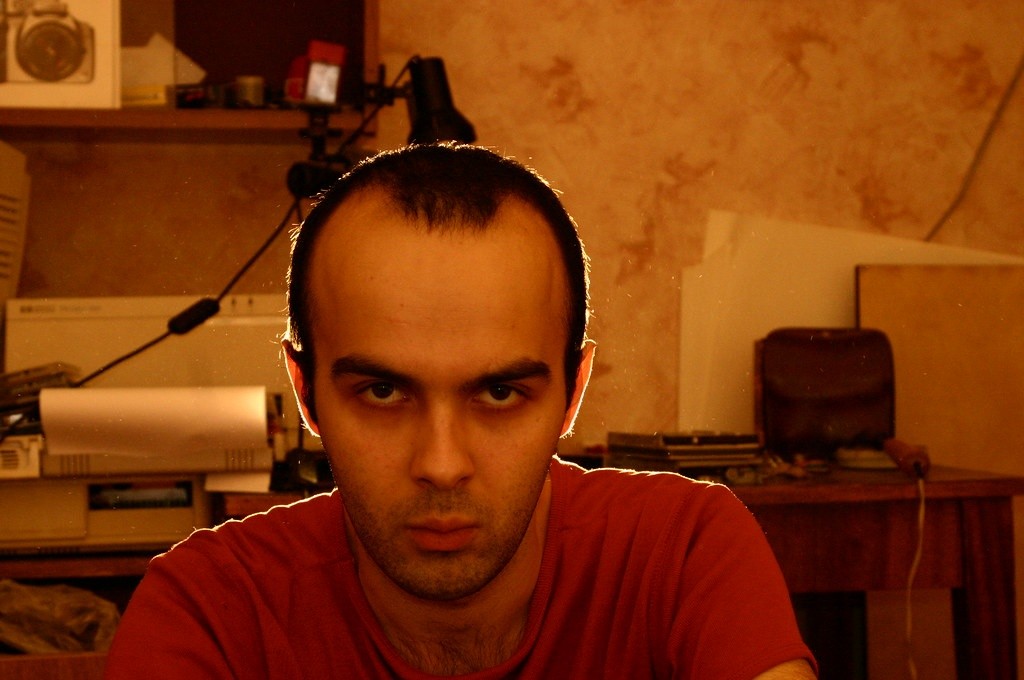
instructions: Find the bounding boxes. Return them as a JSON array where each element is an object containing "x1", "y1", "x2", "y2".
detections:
[{"x1": 285, "y1": 51, "x2": 479, "y2": 201}]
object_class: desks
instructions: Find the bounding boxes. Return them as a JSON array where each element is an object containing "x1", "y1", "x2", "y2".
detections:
[{"x1": 693, "y1": 461, "x2": 1024, "y2": 680}]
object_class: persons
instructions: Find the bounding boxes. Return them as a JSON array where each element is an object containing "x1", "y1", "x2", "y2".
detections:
[{"x1": 102, "y1": 141, "x2": 820, "y2": 680}]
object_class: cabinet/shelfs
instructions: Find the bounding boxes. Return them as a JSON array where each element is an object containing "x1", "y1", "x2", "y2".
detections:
[
  {"x1": 0, "y1": 0, "x2": 379, "y2": 141},
  {"x1": 0, "y1": 472, "x2": 211, "y2": 680}
]
